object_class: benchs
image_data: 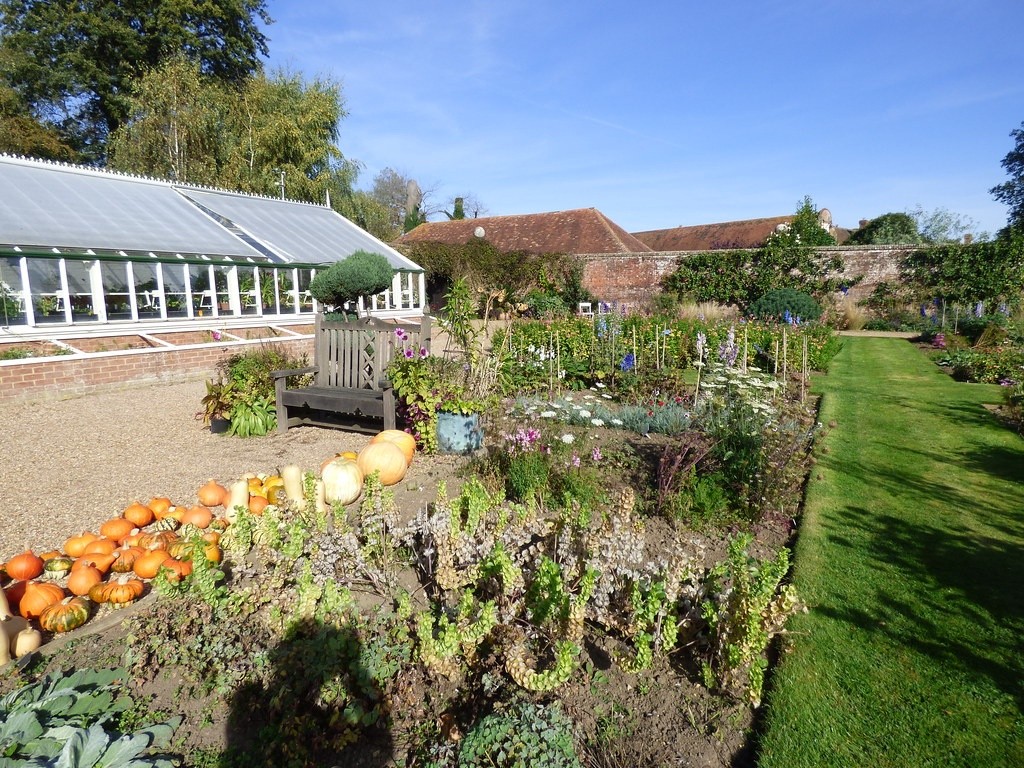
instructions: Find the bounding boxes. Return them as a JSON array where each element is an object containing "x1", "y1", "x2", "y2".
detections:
[{"x1": 267, "y1": 305, "x2": 432, "y2": 436}]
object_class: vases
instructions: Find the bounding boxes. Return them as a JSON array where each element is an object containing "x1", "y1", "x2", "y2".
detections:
[
  {"x1": 435, "y1": 413, "x2": 484, "y2": 454},
  {"x1": 217, "y1": 301, "x2": 221, "y2": 309}
]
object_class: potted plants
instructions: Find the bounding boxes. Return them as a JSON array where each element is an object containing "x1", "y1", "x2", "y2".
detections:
[
  {"x1": 258, "y1": 269, "x2": 275, "y2": 309},
  {"x1": 200, "y1": 378, "x2": 237, "y2": 433},
  {"x1": 221, "y1": 288, "x2": 232, "y2": 310},
  {"x1": 238, "y1": 272, "x2": 253, "y2": 309}
]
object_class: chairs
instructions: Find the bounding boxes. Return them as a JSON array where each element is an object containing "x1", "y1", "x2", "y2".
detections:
[
  {"x1": 579, "y1": 302, "x2": 594, "y2": 320},
  {"x1": 598, "y1": 302, "x2": 612, "y2": 315}
]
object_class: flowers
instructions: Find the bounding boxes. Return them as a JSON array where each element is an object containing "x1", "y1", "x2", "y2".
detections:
[{"x1": 386, "y1": 321, "x2": 485, "y2": 456}]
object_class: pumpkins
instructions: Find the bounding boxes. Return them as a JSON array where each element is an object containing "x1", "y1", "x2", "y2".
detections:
[{"x1": 0, "y1": 431, "x2": 415, "y2": 667}]
